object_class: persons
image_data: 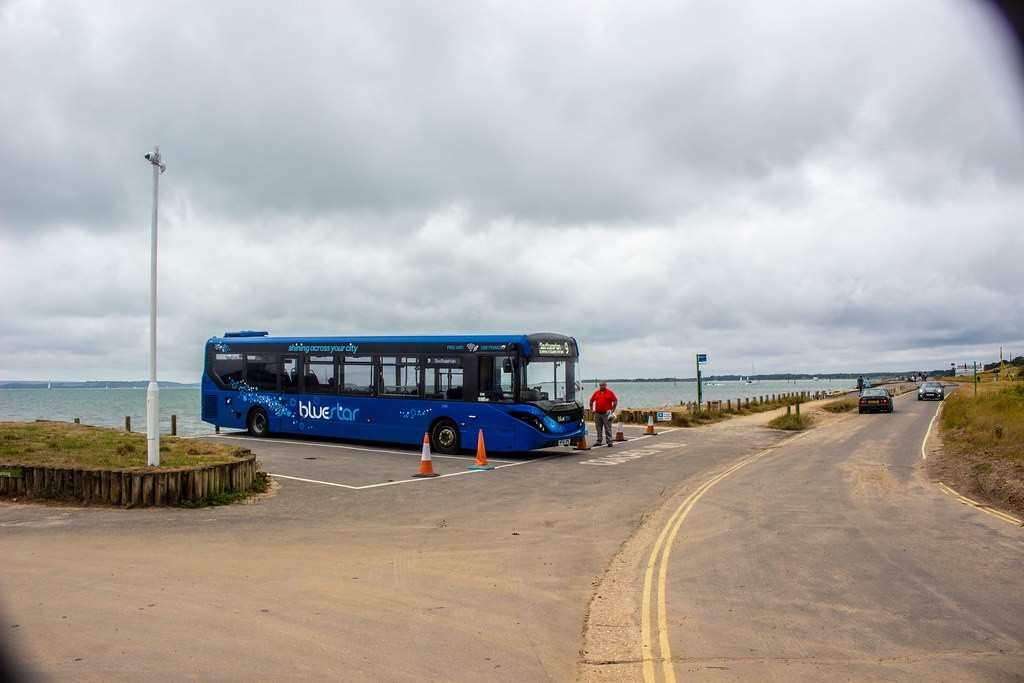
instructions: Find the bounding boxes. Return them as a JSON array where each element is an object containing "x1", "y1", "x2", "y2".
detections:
[
  {"x1": 857, "y1": 376, "x2": 871, "y2": 393},
  {"x1": 560, "y1": 383, "x2": 580, "y2": 446},
  {"x1": 912, "y1": 371, "x2": 928, "y2": 381},
  {"x1": 590, "y1": 380, "x2": 618, "y2": 447}
]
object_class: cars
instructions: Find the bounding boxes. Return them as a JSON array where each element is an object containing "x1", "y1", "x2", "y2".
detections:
[
  {"x1": 858, "y1": 387, "x2": 894, "y2": 413},
  {"x1": 917, "y1": 382, "x2": 946, "y2": 401}
]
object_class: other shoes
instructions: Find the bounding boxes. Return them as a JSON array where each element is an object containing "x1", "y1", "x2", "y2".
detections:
[
  {"x1": 607, "y1": 442, "x2": 613, "y2": 446},
  {"x1": 593, "y1": 443, "x2": 600, "y2": 446}
]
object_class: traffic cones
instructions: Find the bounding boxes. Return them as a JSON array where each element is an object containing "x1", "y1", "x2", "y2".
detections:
[
  {"x1": 612, "y1": 415, "x2": 628, "y2": 442},
  {"x1": 572, "y1": 435, "x2": 592, "y2": 450},
  {"x1": 642, "y1": 410, "x2": 657, "y2": 435},
  {"x1": 411, "y1": 432, "x2": 441, "y2": 477},
  {"x1": 467, "y1": 427, "x2": 495, "y2": 470}
]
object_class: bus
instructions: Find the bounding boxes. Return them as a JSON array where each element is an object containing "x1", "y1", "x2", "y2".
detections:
[{"x1": 200, "y1": 330, "x2": 588, "y2": 456}]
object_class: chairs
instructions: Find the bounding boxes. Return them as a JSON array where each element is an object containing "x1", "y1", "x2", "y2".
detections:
[
  {"x1": 283, "y1": 368, "x2": 320, "y2": 390},
  {"x1": 879, "y1": 391, "x2": 883, "y2": 395},
  {"x1": 866, "y1": 391, "x2": 872, "y2": 395}
]
